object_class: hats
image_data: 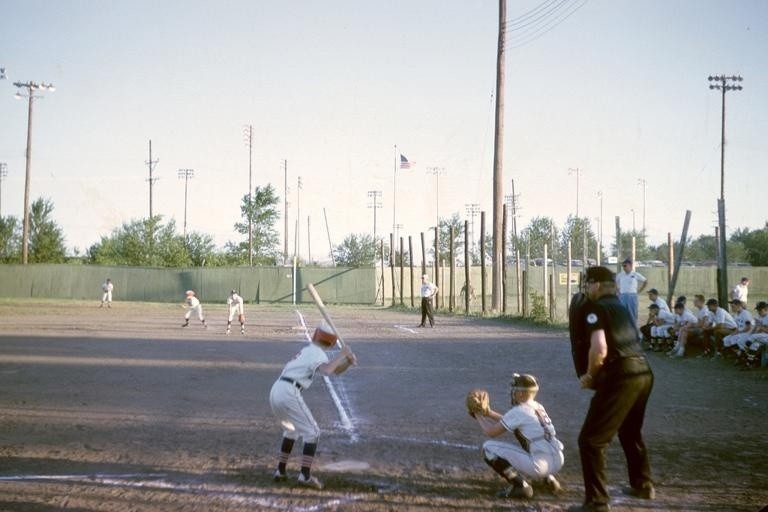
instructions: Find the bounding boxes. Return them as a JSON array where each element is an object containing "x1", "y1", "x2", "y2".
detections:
[
  {"x1": 649, "y1": 304, "x2": 658, "y2": 308},
  {"x1": 647, "y1": 288, "x2": 657, "y2": 294},
  {"x1": 728, "y1": 300, "x2": 739, "y2": 304},
  {"x1": 705, "y1": 299, "x2": 716, "y2": 305},
  {"x1": 622, "y1": 259, "x2": 631, "y2": 264}
]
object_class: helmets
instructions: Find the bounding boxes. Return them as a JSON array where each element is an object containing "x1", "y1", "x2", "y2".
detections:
[
  {"x1": 313, "y1": 326, "x2": 337, "y2": 346},
  {"x1": 510, "y1": 372, "x2": 540, "y2": 405},
  {"x1": 580, "y1": 267, "x2": 614, "y2": 297}
]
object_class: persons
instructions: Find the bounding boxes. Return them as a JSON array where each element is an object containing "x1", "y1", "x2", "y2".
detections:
[
  {"x1": 567, "y1": 266, "x2": 654, "y2": 512},
  {"x1": 615, "y1": 259, "x2": 646, "y2": 342},
  {"x1": 459, "y1": 281, "x2": 476, "y2": 308},
  {"x1": 270, "y1": 326, "x2": 353, "y2": 491},
  {"x1": 182, "y1": 290, "x2": 208, "y2": 330},
  {"x1": 472, "y1": 373, "x2": 565, "y2": 500},
  {"x1": 729, "y1": 277, "x2": 749, "y2": 309},
  {"x1": 418, "y1": 274, "x2": 438, "y2": 328},
  {"x1": 639, "y1": 288, "x2": 767, "y2": 371},
  {"x1": 97, "y1": 278, "x2": 114, "y2": 307},
  {"x1": 223, "y1": 289, "x2": 245, "y2": 335}
]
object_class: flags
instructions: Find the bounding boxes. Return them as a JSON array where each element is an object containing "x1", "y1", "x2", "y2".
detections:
[{"x1": 399, "y1": 153, "x2": 417, "y2": 170}]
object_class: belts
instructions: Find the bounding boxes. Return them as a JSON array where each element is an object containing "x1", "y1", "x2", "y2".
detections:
[{"x1": 280, "y1": 377, "x2": 301, "y2": 388}]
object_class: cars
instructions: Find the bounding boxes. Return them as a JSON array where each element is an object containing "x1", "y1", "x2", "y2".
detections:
[{"x1": 505, "y1": 254, "x2": 754, "y2": 274}]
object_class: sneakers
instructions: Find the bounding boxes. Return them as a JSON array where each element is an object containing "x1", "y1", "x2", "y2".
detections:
[
  {"x1": 298, "y1": 472, "x2": 324, "y2": 489},
  {"x1": 569, "y1": 504, "x2": 609, "y2": 512},
  {"x1": 497, "y1": 486, "x2": 533, "y2": 499},
  {"x1": 550, "y1": 481, "x2": 561, "y2": 492},
  {"x1": 649, "y1": 344, "x2": 684, "y2": 359},
  {"x1": 704, "y1": 348, "x2": 753, "y2": 371},
  {"x1": 274, "y1": 470, "x2": 288, "y2": 479},
  {"x1": 622, "y1": 485, "x2": 655, "y2": 500}
]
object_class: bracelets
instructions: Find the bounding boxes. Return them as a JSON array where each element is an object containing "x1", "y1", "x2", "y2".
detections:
[
  {"x1": 431, "y1": 294, "x2": 433, "y2": 297},
  {"x1": 585, "y1": 373, "x2": 594, "y2": 379}
]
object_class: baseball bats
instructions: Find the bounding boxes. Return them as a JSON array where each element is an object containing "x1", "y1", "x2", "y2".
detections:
[{"x1": 306, "y1": 284, "x2": 356, "y2": 368}]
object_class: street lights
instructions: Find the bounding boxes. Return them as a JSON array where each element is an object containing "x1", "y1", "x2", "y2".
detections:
[
  {"x1": 0, "y1": 160, "x2": 10, "y2": 180},
  {"x1": 11, "y1": 76, "x2": 58, "y2": 263},
  {"x1": 705, "y1": 68, "x2": 742, "y2": 317}
]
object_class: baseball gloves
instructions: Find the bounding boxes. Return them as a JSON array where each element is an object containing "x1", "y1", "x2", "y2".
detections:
[{"x1": 467, "y1": 389, "x2": 490, "y2": 418}]
